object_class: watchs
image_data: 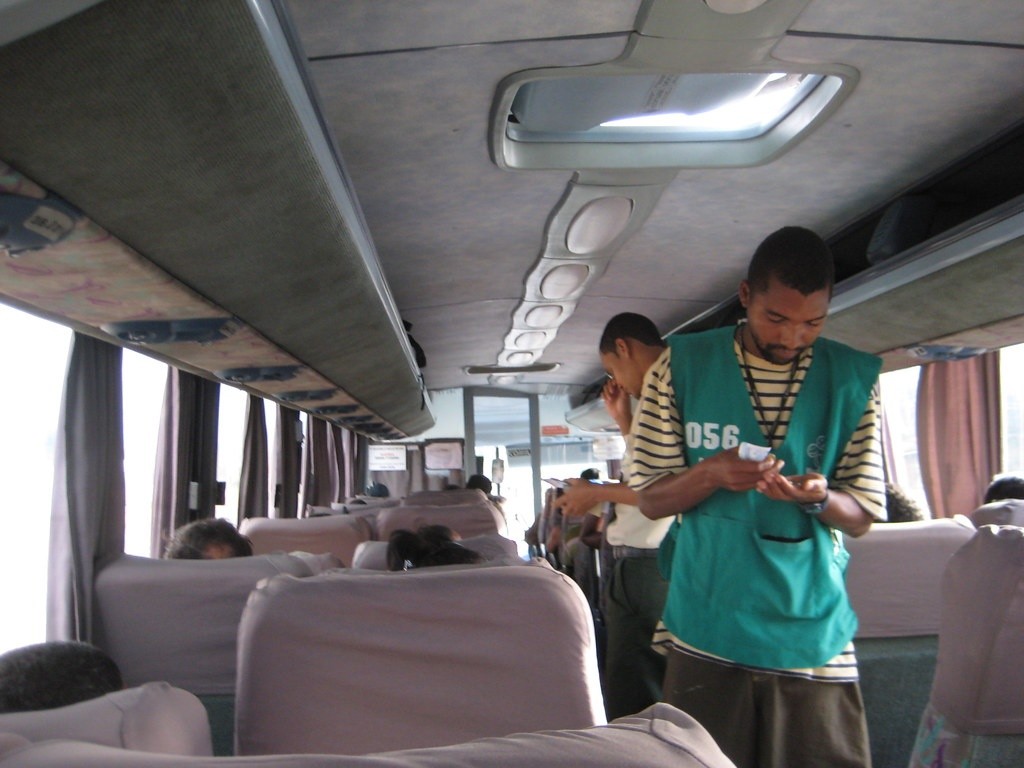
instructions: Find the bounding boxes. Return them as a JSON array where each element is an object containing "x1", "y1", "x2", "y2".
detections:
[{"x1": 799, "y1": 491, "x2": 829, "y2": 515}]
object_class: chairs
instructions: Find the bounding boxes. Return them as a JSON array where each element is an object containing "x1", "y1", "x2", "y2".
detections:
[{"x1": 0, "y1": 488, "x2": 1024, "y2": 768}]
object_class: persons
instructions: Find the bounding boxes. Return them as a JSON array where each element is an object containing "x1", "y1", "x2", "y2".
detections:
[
  {"x1": 628, "y1": 226, "x2": 888, "y2": 768},
  {"x1": 387, "y1": 524, "x2": 489, "y2": 571},
  {"x1": 984, "y1": 476, "x2": 1024, "y2": 504},
  {"x1": 368, "y1": 484, "x2": 388, "y2": 497},
  {"x1": 525, "y1": 468, "x2": 619, "y2": 578},
  {"x1": 552, "y1": 311, "x2": 688, "y2": 723},
  {"x1": 885, "y1": 483, "x2": 924, "y2": 522},
  {"x1": 0, "y1": 640, "x2": 128, "y2": 713},
  {"x1": 467, "y1": 474, "x2": 493, "y2": 494},
  {"x1": 163, "y1": 518, "x2": 255, "y2": 560}
]
